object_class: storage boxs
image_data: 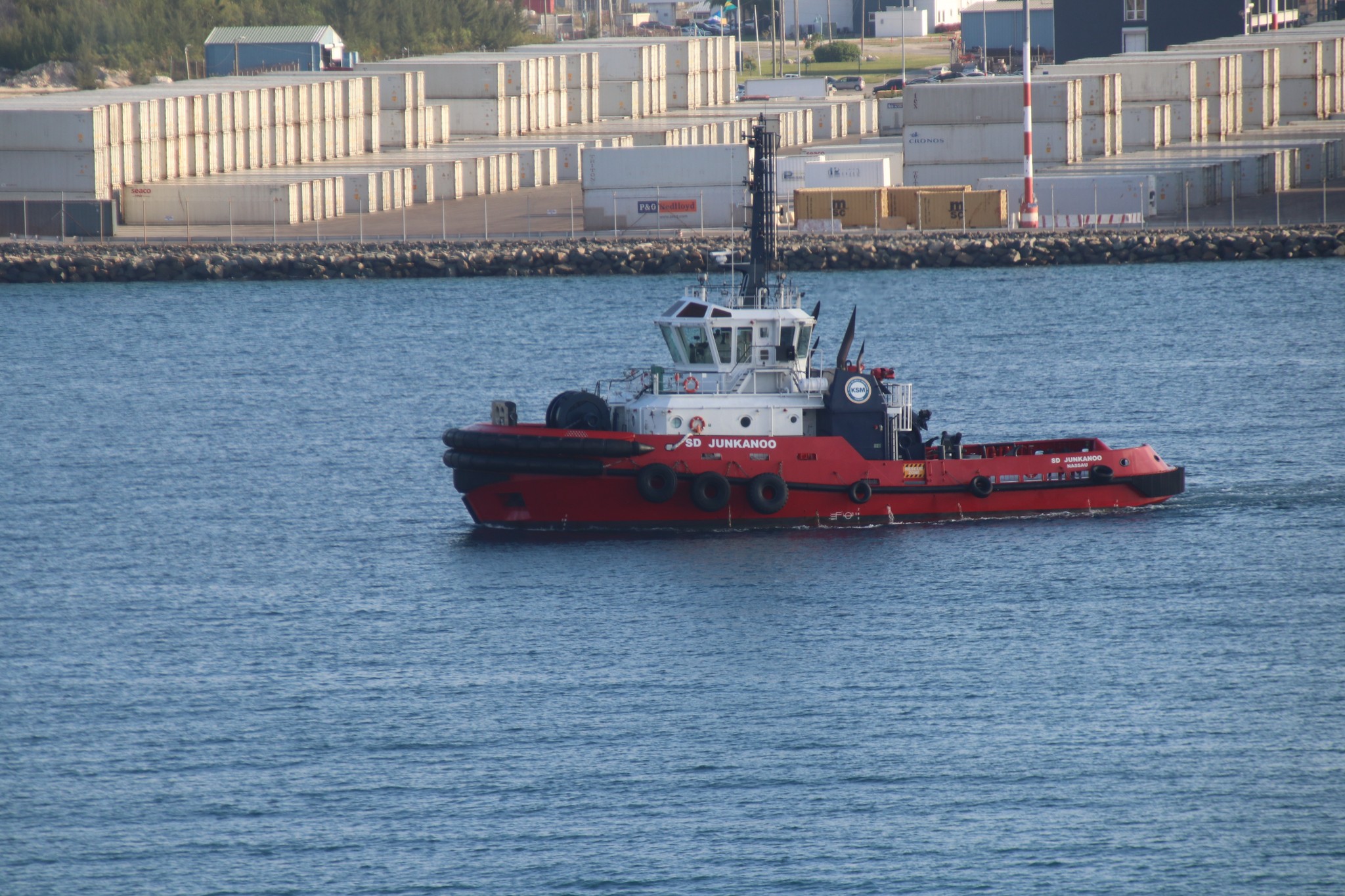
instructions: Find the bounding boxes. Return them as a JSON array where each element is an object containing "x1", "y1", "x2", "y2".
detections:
[{"x1": 0, "y1": 18, "x2": 1345, "y2": 231}]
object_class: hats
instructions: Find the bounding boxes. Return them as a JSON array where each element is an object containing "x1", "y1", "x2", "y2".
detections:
[{"x1": 942, "y1": 431, "x2": 948, "y2": 435}]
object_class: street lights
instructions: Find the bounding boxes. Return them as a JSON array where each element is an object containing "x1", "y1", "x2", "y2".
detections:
[
  {"x1": 814, "y1": 14, "x2": 822, "y2": 46},
  {"x1": 580, "y1": 10, "x2": 589, "y2": 39},
  {"x1": 689, "y1": 19, "x2": 705, "y2": 36}
]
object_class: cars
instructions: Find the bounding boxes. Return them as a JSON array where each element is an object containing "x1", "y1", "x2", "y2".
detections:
[
  {"x1": 784, "y1": 74, "x2": 802, "y2": 78},
  {"x1": 624, "y1": 19, "x2": 755, "y2": 38},
  {"x1": 905, "y1": 70, "x2": 1023, "y2": 85},
  {"x1": 828, "y1": 76, "x2": 865, "y2": 92},
  {"x1": 873, "y1": 78, "x2": 906, "y2": 95}
]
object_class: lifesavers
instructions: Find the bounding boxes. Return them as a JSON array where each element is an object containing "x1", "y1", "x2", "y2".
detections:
[
  {"x1": 1026, "y1": 474, "x2": 1038, "y2": 478},
  {"x1": 748, "y1": 473, "x2": 788, "y2": 513},
  {"x1": 1093, "y1": 465, "x2": 1113, "y2": 480},
  {"x1": 972, "y1": 475, "x2": 994, "y2": 496},
  {"x1": 850, "y1": 482, "x2": 872, "y2": 503},
  {"x1": 690, "y1": 416, "x2": 705, "y2": 432},
  {"x1": 637, "y1": 463, "x2": 678, "y2": 503},
  {"x1": 683, "y1": 377, "x2": 699, "y2": 394},
  {"x1": 691, "y1": 471, "x2": 732, "y2": 512}
]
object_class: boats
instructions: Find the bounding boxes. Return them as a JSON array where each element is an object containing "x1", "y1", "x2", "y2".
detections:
[{"x1": 443, "y1": 112, "x2": 1186, "y2": 529}]
